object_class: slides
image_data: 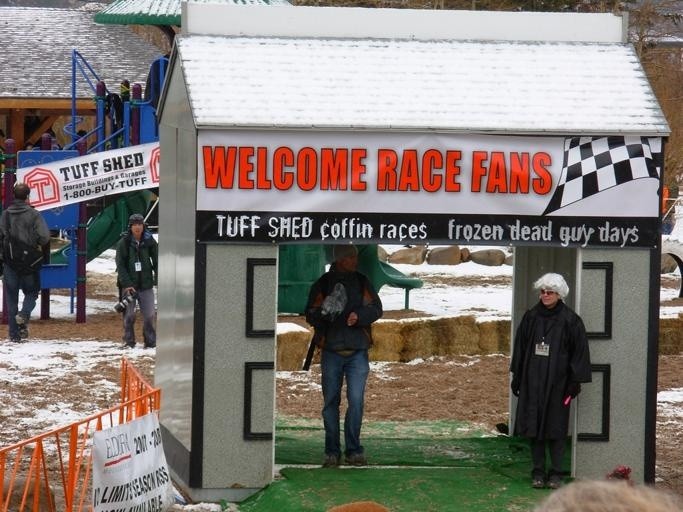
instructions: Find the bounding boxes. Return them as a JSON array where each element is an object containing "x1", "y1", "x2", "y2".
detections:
[{"x1": 51, "y1": 190, "x2": 151, "y2": 267}]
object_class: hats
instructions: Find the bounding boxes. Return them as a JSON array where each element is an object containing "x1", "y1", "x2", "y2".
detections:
[
  {"x1": 534, "y1": 272, "x2": 570, "y2": 298},
  {"x1": 128, "y1": 214, "x2": 144, "y2": 224}
]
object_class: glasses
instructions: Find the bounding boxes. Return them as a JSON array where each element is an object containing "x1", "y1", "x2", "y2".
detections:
[{"x1": 540, "y1": 289, "x2": 558, "y2": 295}]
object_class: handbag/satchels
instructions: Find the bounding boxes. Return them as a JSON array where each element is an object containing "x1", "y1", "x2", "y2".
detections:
[{"x1": 2, "y1": 212, "x2": 44, "y2": 274}]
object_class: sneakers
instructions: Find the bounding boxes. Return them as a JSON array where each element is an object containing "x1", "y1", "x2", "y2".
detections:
[
  {"x1": 8, "y1": 311, "x2": 29, "y2": 343},
  {"x1": 323, "y1": 453, "x2": 366, "y2": 468},
  {"x1": 531, "y1": 471, "x2": 562, "y2": 488}
]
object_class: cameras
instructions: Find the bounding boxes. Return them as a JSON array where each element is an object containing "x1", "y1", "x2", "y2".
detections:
[{"x1": 114, "y1": 290, "x2": 139, "y2": 314}]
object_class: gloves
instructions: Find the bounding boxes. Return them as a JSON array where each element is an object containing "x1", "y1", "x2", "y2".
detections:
[
  {"x1": 567, "y1": 382, "x2": 581, "y2": 398},
  {"x1": 511, "y1": 374, "x2": 522, "y2": 397}
]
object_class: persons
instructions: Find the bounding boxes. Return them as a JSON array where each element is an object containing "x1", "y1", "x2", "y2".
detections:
[
  {"x1": 0, "y1": 183, "x2": 50, "y2": 342},
  {"x1": 305, "y1": 245, "x2": 383, "y2": 466},
  {"x1": 510, "y1": 272, "x2": 593, "y2": 488},
  {"x1": 116, "y1": 214, "x2": 158, "y2": 349}
]
